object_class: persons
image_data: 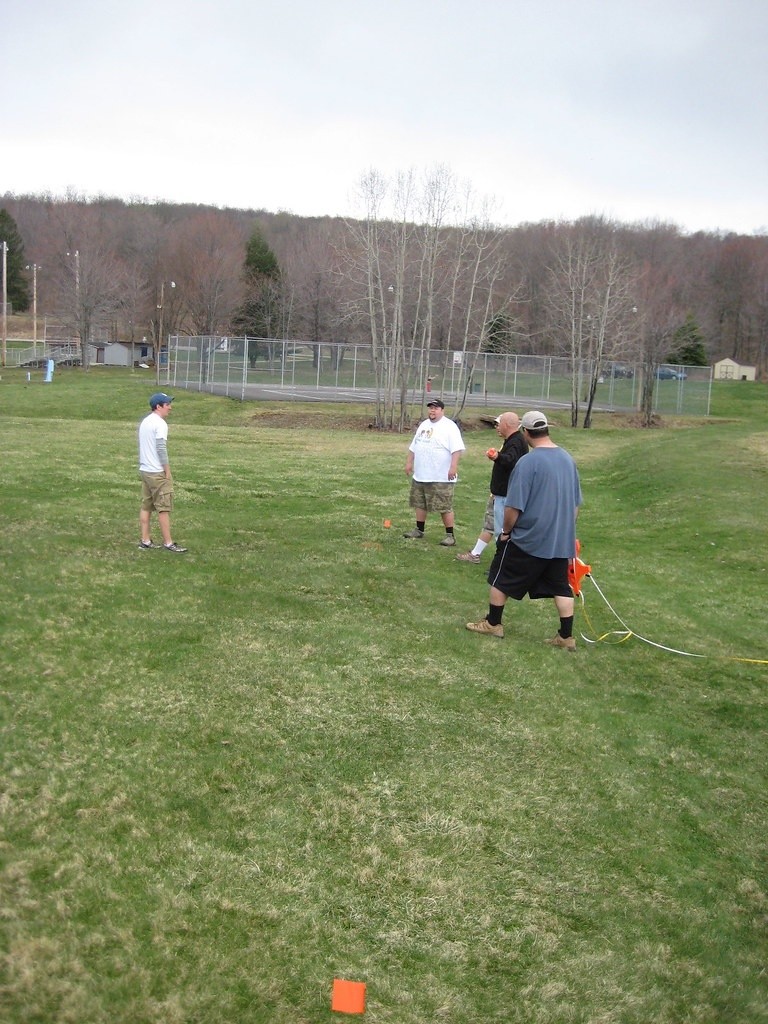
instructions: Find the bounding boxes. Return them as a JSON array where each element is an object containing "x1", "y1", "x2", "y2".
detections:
[
  {"x1": 138, "y1": 393, "x2": 189, "y2": 555},
  {"x1": 402, "y1": 399, "x2": 466, "y2": 547},
  {"x1": 464, "y1": 411, "x2": 584, "y2": 651},
  {"x1": 456, "y1": 411, "x2": 528, "y2": 567}
]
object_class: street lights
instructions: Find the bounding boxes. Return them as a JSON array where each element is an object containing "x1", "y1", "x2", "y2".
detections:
[
  {"x1": 158, "y1": 281, "x2": 178, "y2": 366},
  {"x1": 23, "y1": 261, "x2": 44, "y2": 357},
  {"x1": 388, "y1": 284, "x2": 409, "y2": 371},
  {"x1": 66, "y1": 248, "x2": 82, "y2": 363}
]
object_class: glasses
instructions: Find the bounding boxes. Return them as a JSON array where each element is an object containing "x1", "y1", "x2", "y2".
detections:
[{"x1": 494, "y1": 425, "x2": 497, "y2": 428}]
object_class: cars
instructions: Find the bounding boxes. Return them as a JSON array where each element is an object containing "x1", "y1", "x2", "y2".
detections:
[
  {"x1": 655, "y1": 366, "x2": 689, "y2": 379},
  {"x1": 600, "y1": 362, "x2": 634, "y2": 380}
]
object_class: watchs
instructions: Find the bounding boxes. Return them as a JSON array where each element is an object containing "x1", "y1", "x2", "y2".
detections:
[{"x1": 501, "y1": 528, "x2": 511, "y2": 536}]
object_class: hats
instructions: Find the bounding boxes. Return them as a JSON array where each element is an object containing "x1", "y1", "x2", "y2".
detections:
[
  {"x1": 150, "y1": 392, "x2": 174, "y2": 406},
  {"x1": 427, "y1": 399, "x2": 444, "y2": 409},
  {"x1": 523, "y1": 411, "x2": 548, "y2": 430},
  {"x1": 494, "y1": 415, "x2": 502, "y2": 423}
]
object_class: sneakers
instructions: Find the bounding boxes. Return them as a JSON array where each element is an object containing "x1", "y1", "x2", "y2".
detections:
[
  {"x1": 163, "y1": 542, "x2": 188, "y2": 553},
  {"x1": 544, "y1": 633, "x2": 577, "y2": 652},
  {"x1": 441, "y1": 533, "x2": 456, "y2": 546},
  {"x1": 138, "y1": 540, "x2": 162, "y2": 550},
  {"x1": 455, "y1": 550, "x2": 481, "y2": 564},
  {"x1": 467, "y1": 620, "x2": 503, "y2": 638},
  {"x1": 403, "y1": 528, "x2": 423, "y2": 538}
]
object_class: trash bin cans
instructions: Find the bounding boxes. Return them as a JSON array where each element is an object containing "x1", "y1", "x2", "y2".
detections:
[{"x1": 474, "y1": 384, "x2": 481, "y2": 392}]
